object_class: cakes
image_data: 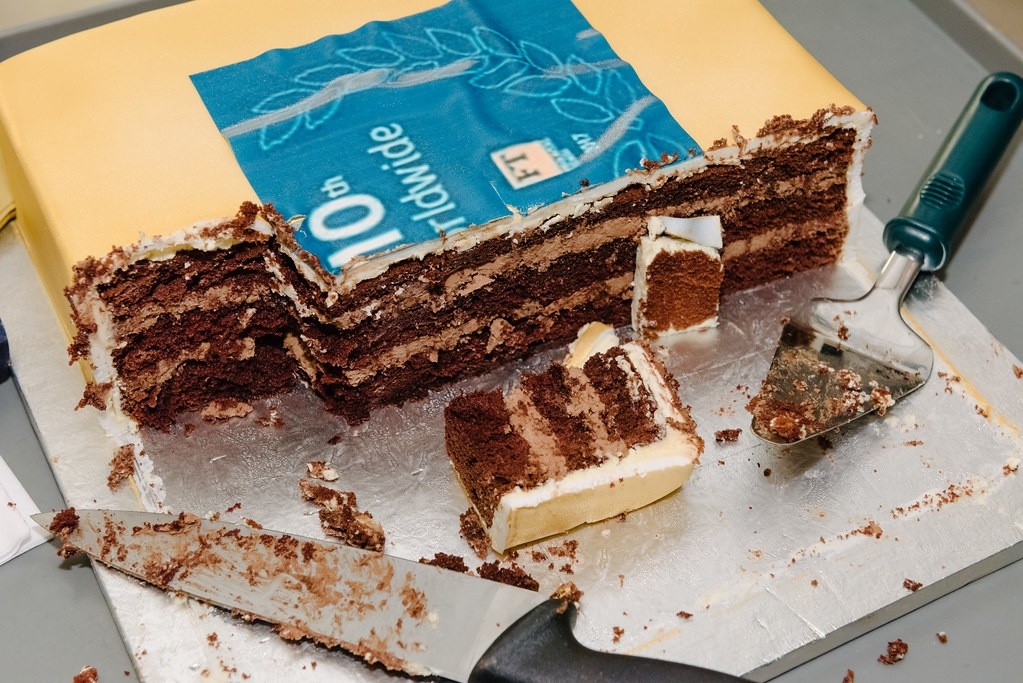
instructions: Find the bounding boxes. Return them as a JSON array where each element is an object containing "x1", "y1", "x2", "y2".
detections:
[{"x1": 2, "y1": 2, "x2": 885, "y2": 553}]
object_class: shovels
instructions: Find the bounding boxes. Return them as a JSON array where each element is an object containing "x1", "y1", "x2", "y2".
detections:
[{"x1": 752, "y1": 72, "x2": 1023, "y2": 446}]
